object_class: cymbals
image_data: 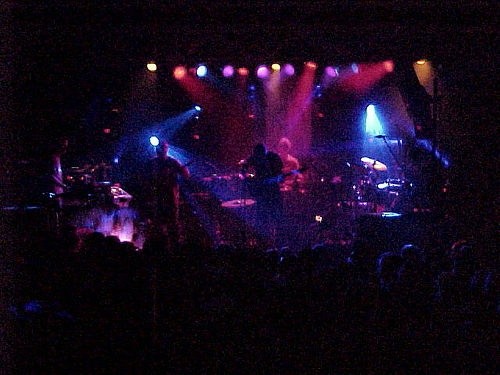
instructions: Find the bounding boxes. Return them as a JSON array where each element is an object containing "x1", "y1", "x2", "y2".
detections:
[{"x1": 362, "y1": 157, "x2": 387, "y2": 172}]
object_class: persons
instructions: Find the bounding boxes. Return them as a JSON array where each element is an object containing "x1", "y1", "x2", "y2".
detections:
[
  {"x1": 32, "y1": 132, "x2": 68, "y2": 240},
  {"x1": 387, "y1": 148, "x2": 445, "y2": 247},
  {"x1": 133, "y1": 139, "x2": 190, "y2": 252},
  {"x1": 243, "y1": 143, "x2": 285, "y2": 243},
  {"x1": 1, "y1": 233, "x2": 500, "y2": 375}
]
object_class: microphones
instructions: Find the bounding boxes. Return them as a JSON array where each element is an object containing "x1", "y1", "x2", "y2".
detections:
[{"x1": 374, "y1": 135, "x2": 390, "y2": 139}]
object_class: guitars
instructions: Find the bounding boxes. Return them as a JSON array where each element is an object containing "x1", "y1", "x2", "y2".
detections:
[
  {"x1": 247, "y1": 166, "x2": 308, "y2": 197},
  {"x1": 144, "y1": 158, "x2": 196, "y2": 208}
]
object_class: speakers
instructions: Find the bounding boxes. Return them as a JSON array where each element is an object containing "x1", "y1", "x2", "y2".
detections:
[{"x1": 358, "y1": 212, "x2": 415, "y2": 249}]
